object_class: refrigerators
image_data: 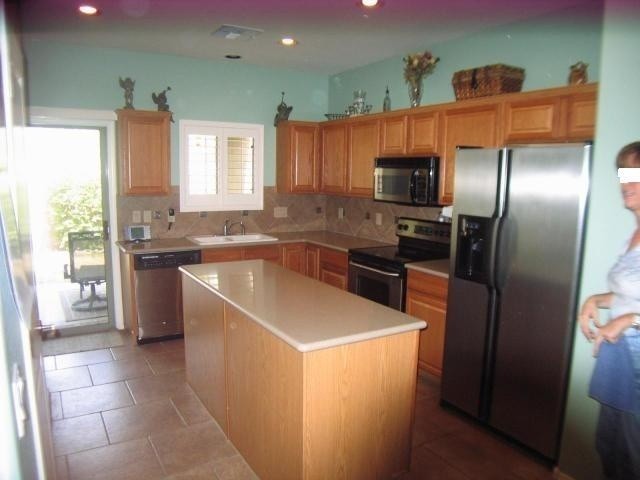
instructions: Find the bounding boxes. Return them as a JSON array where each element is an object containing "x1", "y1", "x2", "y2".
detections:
[{"x1": 440, "y1": 146, "x2": 591, "y2": 462}]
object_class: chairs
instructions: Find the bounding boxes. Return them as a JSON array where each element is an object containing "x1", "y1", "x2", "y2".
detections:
[{"x1": 64, "y1": 231, "x2": 104, "y2": 311}]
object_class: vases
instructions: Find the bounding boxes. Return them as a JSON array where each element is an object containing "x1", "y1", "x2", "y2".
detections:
[{"x1": 407, "y1": 78, "x2": 423, "y2": 107}]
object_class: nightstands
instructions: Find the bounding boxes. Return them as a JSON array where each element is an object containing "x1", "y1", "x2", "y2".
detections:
[{"x1": 408, "y1": 103, "x2": 438, "y2": 152}]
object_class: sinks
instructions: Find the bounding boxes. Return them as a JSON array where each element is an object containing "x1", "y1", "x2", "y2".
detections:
[
  {"x1": 227, "y1": 235, "x2": 272, "y2": 241},
  {"x1": 195, "y1": 237, "x2": 233, "y2": 244}
]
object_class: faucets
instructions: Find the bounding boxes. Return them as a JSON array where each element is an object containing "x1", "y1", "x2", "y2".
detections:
[
  {"x1": 228, "y1": 222, "x2": 241, "y2": 234},
  {"x1": 240, "y1": 221, "x2": 245, "y2": 234}
]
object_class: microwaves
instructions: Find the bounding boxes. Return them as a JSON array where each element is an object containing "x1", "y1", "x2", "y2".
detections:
[{"x1": 374, "y1": 159, "x2": 436, "y2": 207}]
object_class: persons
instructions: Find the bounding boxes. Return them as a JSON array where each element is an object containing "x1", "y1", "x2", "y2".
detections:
[{"x1": 578, "y1": 142, "x2": 640, "y2": 479}]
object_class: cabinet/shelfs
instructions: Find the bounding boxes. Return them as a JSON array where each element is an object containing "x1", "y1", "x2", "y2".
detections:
[
  {"x1": 440, "y1": 97, "x2": 501, "y2": 205},
  {"x1": 284, "y1": 243, "x2": 306, "y2": 274},
  {"x1": 113, "y1": 109, "x2": 172, "y2": 194},
  {"x1": 406, "y1": 268, "x2": 448, "y2": 385},
  {"x1": 318, "y1": 117, "x2": 380, "y2": 197},
  {"x1": 306, "y1": 242, "x2": 348, "y2": 289},
  {"x1": 502, "y1": 86, "x2": 561, "y2": 144},
  {"x1": 561, "y1": 81, "x2": 598, "y2": 143},
  {"x1": 381, "y1": 112, "x2": 407, "y2": 156},
  {"x1": 275, "y1": 120, "x2": 318, "y2": 193}
]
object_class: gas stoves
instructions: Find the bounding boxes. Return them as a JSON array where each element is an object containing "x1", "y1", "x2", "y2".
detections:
[{"x1": 351, "y1": 243, "x2": 440, "y2": 263}]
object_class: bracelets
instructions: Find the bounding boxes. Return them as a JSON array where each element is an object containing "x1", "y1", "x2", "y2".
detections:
[{"x1": 633, "y1": 313, "x2": 640, "y2": 328}]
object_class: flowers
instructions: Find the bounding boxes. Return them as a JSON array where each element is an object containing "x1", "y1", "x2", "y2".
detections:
[{"x1": 403, "y1": 53, "x2": 438, "y2": 102}]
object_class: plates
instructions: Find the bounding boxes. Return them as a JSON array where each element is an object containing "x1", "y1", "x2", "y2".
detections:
[{"x1": 324, "y1": 113, "x2": 350, "y2": 120}]
